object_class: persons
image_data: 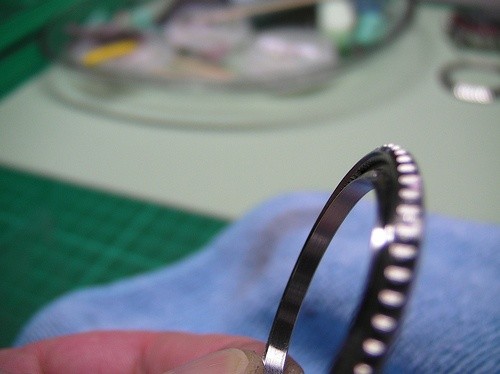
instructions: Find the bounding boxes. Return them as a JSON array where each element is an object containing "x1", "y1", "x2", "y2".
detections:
[{"x1": 0, "y1": 329, "x2": 306, "y2": 374}]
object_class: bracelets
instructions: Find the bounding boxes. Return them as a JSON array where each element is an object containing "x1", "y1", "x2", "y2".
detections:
[
  {"x1": 261, "y1": 139, "x2": 426, "y2": 374},
  {"x1": 439, "y1": 56, "x2": 499, "y2": 105}
]
